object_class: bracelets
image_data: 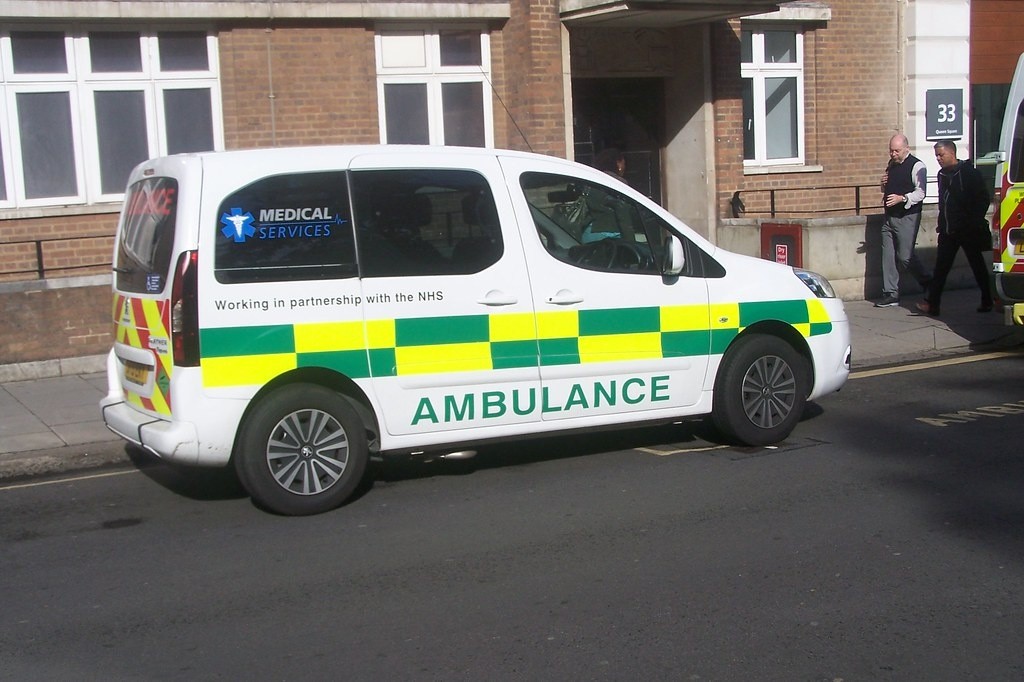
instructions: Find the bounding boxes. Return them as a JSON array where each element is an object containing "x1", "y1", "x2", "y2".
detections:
[{"x1": 902, "y1": 195, "x2": 907, "y2": 201}]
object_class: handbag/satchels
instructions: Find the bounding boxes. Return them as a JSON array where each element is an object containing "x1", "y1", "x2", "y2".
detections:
[{"x1": 971, "y1": 219, "x2": 994, "y2": 252}]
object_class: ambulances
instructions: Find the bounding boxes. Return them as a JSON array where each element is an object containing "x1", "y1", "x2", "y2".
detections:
[
  {"x1": 99, "y1": 144, "x2": 852, "y2": 516},
  {"x1": 991, "y1": 52, "x2": 1024, "y2": 329}
]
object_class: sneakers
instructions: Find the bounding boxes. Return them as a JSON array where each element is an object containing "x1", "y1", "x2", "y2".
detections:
[
  {"x1": 875, "y1": 295, "x2": 899, "y2": 308},
  {"x1": 977, "y1": 298, "x2": 1000, "y2": 312},
  {"x1": 915, "y1": 302, "x2": 940, "y2": 317}
]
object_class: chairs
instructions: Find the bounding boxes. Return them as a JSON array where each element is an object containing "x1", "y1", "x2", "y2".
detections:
[
  {"x1": 382, "y1": 192, "x2": 453, "y2": 273},
  {"x1": 455, "y1": 195, "x2": 500, "y2": 272}
]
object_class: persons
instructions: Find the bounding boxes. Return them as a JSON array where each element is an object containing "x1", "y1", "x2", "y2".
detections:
[
  {"x1": 919, "y1": 141, "x2": 995, "y2": 318},
  {"x1": 876, "y1": 135, "x2": 926, "y2": 307},
  {"x1": 581, "y1": 148, "x2": 636, "y2": 241}
]
